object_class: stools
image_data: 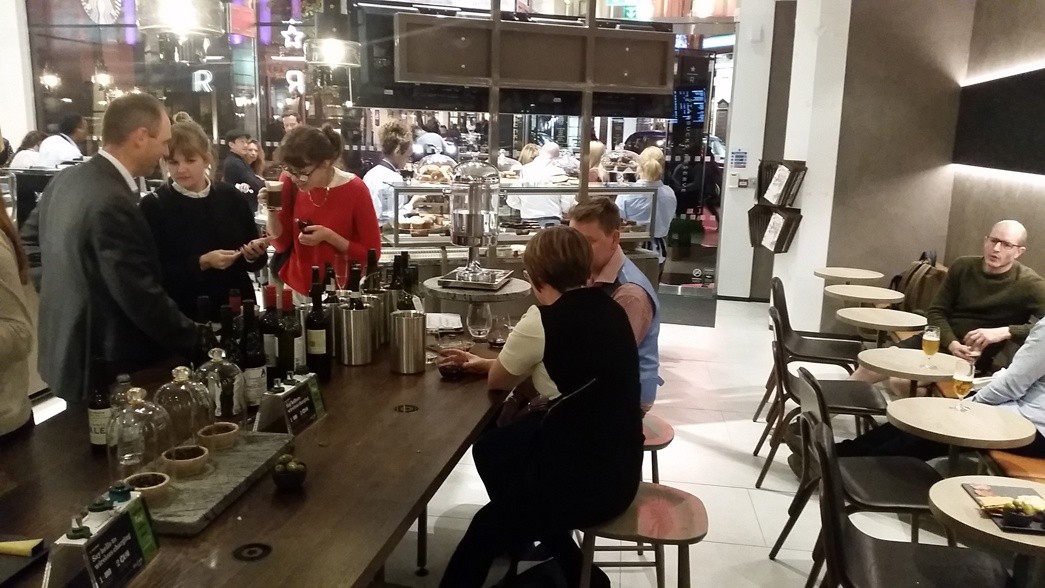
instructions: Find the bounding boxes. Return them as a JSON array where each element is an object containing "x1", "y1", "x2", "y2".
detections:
[
  {"x1": 636, "y1": 412, "x2": 675, "y2": 556},
  {"x1": 579, "y1": 481, "x2": 709, "y2": 588}
]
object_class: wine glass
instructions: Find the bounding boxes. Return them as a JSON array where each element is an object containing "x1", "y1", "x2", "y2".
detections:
[
  {"x1": 952, "y1": 360, "x2": 974, "y2": 412},
  {"x1": 922, "y1": 326, "x2": 940, "y2": 367}
]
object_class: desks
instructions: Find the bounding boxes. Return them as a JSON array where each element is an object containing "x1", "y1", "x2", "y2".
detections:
[
  {"x1": 834, "y1": 307, "x2": 928, "y2": 348},
  {"x1": 813, "y1": 265, "x2": 886, "y2": 308},
  {"x1": 886, "y1": 396, "x2": 1037, "y2": 475},
  {"x1": 858, "y1": 347, "x2": 972, "y2": 398},
  {"x1": 823, "y1": 284, "x2": 906, "y2": 348},
  {"x1": 928, "y1": 476, "x2": 1045, "y2": 588},
  {"x1": 0, "y1": 319, "x2": 521, "y2": 588}
]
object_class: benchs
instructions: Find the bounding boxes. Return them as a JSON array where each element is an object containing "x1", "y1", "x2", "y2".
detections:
[{"x1": 932, "y1": 380, "x2": 1045, "y2": 484}]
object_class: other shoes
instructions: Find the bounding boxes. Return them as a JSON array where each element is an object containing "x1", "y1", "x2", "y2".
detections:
[
  {"x1": 784, "y1": 427, "x2": 805, "y2": 458},
  {"x1": 787, "y1": 456, "x2": 803, "y2": 479}
]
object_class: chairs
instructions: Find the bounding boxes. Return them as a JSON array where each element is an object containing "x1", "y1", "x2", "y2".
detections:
[
  {"x1": 768, "y1": 366, "x2": 959, "y2": 588},
  {"x1": 752, "y1": 306, "x2": 889, "y2": 490},
  {"x1": 752, "y1": 277, "x2": 867, "y2": 423},
  {"x1": 811, "y1": 423, "x2": 1015, "y2": 588}
]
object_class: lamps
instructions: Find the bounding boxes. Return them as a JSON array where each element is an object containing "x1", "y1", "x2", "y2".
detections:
[
  {"x1": 303, "y1": 0, "x2": 363, "y2": 69},
  {"x1": 134, "y1": 0, "x2": 227, "y2": 40}
]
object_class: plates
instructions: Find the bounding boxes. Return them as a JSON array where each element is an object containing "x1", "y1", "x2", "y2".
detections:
[{"x1": 961, "y1": 483, "x2": 1045, "y2": 534}]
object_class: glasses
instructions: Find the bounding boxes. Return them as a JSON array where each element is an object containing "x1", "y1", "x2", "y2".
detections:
[
  {"x1": 281, "y1": 162, "x2": 324, "y2": 183},
  {"x1": 986, "y1": 233, "x2": 1022, "y2": 251}
]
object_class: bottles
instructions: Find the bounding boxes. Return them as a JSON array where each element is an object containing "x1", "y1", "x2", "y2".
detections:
[
  {"x1": 88, "y1": 386, "x2": 112, "y2": 458},
  {"x1": 110, "y1": 374, "x2": 134, "y2": 457},
  {"x1": 219, "y1": 305, "x2": 242, "y2": 373},
  {"x1": 229, "y1": 284, "x2": 305, "y2": 422},
  {"x1": 304, "y1": 264, "x2": 333, "y2": 378},
  {"x1": 322, "y1": 248, "x2": 419, "y2": 312},
  {"x1": 191, "y1": 295, "x2": 219, "y2": 371}
]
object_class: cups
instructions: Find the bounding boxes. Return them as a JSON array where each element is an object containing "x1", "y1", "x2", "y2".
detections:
[
  {"x1": 262, "y1": 181, "x2": 284, "y2": 211},
  {"x1": 437, "y1": 341, "x2": 467, "y2": 379},
  {"x1": 296, "y1": 286, "x2": 426, "y2": 375},
  {"x1": 486, "y1": 312, "x2": 511, "y2": 347},
  {"x1": 466, "y1": 303, "x2": 493, "y2": 337},
  {"x1": 425, "y1": 328, "x2": 438, "y2": 364}
]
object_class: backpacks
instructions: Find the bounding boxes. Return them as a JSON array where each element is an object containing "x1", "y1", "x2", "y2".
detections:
[{"x1": 889, "y1": 250, "x2": 949, "y2": 313}]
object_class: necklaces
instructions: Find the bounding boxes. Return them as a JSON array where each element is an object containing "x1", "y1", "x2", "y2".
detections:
[{"x1": 304, "y1": 184, "x2": 330, "y2": 213}]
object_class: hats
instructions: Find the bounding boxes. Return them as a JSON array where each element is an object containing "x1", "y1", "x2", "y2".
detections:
[{"x1": 225, "y1": 130, "x2": 251, "y2": 144}]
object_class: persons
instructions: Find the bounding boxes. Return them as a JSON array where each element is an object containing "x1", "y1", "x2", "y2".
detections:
[
  {"x1": 778, "y1": 219, "x2": 1045, "y2": 491},
  {"x1": 0, "y1": 92, "x2": 307, "y2": 448},
  {"x1": 356, "y1": 120, "x2": 428, "y2": 228},
  {"x1": 508, "y1": 136, "x2": 678, "y2": 295},
  {"x1": 433, "y1": 197, "x2": 664, "y2": 588},
  {"x1": 257, "y1": 123, "x2": 382, "y2": 308}
]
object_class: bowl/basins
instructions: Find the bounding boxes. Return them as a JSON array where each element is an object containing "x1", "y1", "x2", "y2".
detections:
[
  {"x1": 602, "y1": 156, "x2": 638, "y2": 172},
  {"x1": 1002, "y1": 508, "x2": 1035, "y2": 527}
]
